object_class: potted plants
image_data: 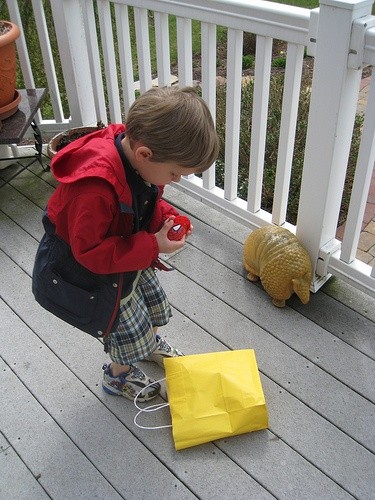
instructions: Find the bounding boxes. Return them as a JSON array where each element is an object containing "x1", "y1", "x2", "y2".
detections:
[{"x1": 47, "y1": 120, "x2": 107, "y2": 159}]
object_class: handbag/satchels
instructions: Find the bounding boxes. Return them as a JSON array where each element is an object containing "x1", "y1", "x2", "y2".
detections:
[{"x1": 134, "y1": 349, "x2": 269, "y2": 451}]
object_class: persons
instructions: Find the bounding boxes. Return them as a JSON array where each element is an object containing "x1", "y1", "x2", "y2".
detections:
[{"x1": 32, "y1": 85, "x2": 219, "y2": 402}]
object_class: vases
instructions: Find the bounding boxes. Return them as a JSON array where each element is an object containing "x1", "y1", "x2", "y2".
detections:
[{"x1": 0, "y1": 20, "x2": 21, "y2": 124}]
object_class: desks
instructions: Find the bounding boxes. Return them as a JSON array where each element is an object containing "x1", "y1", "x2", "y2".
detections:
[{"x1": 0, "y1": 88, "x2": 51, "y2": 171}]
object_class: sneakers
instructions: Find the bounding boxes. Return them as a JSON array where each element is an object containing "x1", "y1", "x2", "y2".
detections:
[
  {"x1": 134, "y1": 335, "x2": 185, "y2": 370},
  {"x1": 102, "y1": 362, "x2": 161, "y2": 403}
]
